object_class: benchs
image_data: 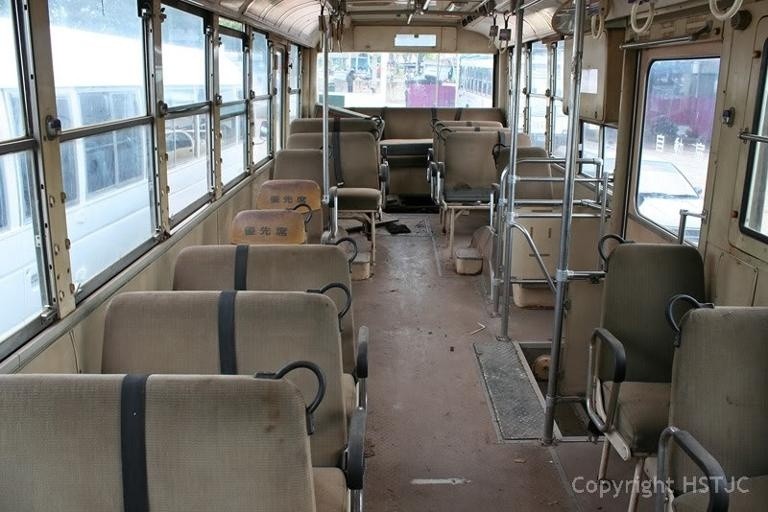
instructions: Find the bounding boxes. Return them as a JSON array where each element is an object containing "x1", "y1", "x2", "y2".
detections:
[{"x1": 0, "y1": 107, "x2": 531, "y2": 512}]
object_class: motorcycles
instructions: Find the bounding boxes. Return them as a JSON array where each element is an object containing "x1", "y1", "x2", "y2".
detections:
[{"x1": 650, "y1": 114, "x2": 691, "y2": 142}]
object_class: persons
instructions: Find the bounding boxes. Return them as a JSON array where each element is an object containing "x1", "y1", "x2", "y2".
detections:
[{"x1": 346, "y1": 71, "x2": 356, "y2": 93}]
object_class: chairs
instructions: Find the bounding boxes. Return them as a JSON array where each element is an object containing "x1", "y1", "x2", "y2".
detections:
[{"x1": 489, "y1": 147, "x2": 767, "y2": 512}]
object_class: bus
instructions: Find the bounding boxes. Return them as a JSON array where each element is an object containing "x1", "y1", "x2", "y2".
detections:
[
  {"x1": 456, "y1": 59, "x2": 577, "y2": 149},
  {"x1": 401, "y1": 61, "x2": 457, "y2": 109},
  {"x1": 0, "y1": 19, "x2": 268, "y2": 341}
]
